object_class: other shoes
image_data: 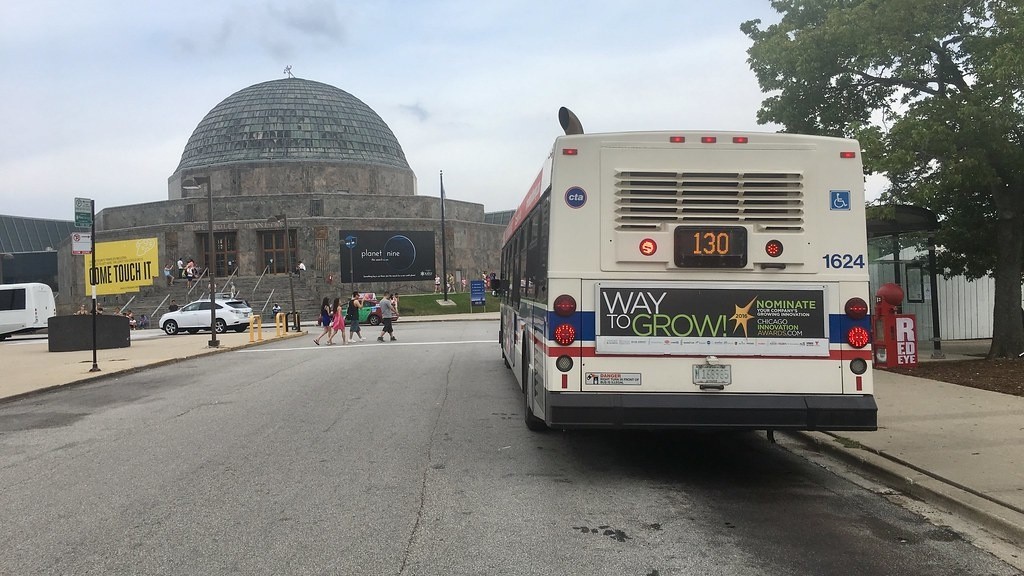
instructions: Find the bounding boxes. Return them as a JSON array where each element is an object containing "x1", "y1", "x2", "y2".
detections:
[
  {"x1": 378, "y1": 337, "x2": 385, "y2": 342},
  {"x1": 313, "y1": 339, "x2": 319, "y2": 345},
  {"x1": 360, "y1": 338, "x2": 366, "y2": 341},
  {"x1": 391, "y1": 336, "x2": 396, "y2": 340},
  {"x1": 348, "y1": 339, "x2": 356, "y2": 342}
]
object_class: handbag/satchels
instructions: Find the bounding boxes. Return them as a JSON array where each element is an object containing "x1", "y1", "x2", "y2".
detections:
[
  {"x1": 347, "y1": 312, "x2": 353, "y2": 320},
  {"x1": 318, "y1": 317, "x2": 323, "y2": 322},
  {"x1": 329, "y1": 320, "x2": 334, "y2": 326}
]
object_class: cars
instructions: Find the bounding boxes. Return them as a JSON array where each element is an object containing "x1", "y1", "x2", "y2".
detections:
[{"x1": 316, "y1": 298, "x2": 400, "y2": 327}]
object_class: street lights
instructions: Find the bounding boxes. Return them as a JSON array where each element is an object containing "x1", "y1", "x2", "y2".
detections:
[
  {"x1": 178, "y1": 174, "x2": 224, "y2": 348},
  {"x1": 265, "y1": 212, "x2": 297, "y2": 332},
  {"x1": 337, "y1": 237, "x2": 354, "y2": 296}
]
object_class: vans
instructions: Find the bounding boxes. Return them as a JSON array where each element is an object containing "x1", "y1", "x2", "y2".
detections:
[{"x1": 0, "y1": 282, "x2": 57, "y2": 341}]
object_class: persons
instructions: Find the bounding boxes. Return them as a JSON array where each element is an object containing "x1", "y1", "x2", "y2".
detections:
[
  {"x1": 169, "y1": 300, "x2": 178, "y2": 311},
  {"x1": 230, "y1": 281, "x2": 235, "y2": 295},
  {"x1": 461, "y1": 278, "x2": 467, "y2": 293},
  {"x1": 73, "y1": 303, "x2": 103, "y2": 315},
  {"x1": 326, "y1": 298, "x2": 348, "y2": 345},
  {"x1": 434, "y1": 274, "x2": 442, "y2": 294},
  {"x1": 296, "y1": 261, "x2": 306, "y2": 277},
  {"x1": 482, "y1": 270, "x2": 496, "y2": 292},
  {"x1": 113, "y1": 308, "x2": 123, "y2": 315},
  {"x1": 347, "y1": 291, "x2": 366, "y2": 343},
  {"x1": 123, "y1": 310, "x2": 148, "y2": 330},
  {"x1": 177, "y1": 258, "x2": 197, "y2": 288},
  {"x1": 377, "y1": 291, "x2": 397, "y2": 342},
  {"x1": 208, "y1": 281, "x2": 217, "y2": 292},
  {"x1": 313, "y1": 297, "x2": 335, "y2": 345},
  {"x1": 164, "y1": 264, "x2": 175, "y2": 286},
  {"x1": 271, "y1": 303, "x2": 281, "y2": 318},
  {"x1": 390, "y1": 293, "x2": 400, "y2": 313},
  {"x1": 446, "y1": 273, "x2": 456, "y2": 294}
]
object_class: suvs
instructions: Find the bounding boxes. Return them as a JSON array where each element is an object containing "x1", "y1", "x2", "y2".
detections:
[{"x1": 158, "y1": 297, "x2": 255, "y2": 334}]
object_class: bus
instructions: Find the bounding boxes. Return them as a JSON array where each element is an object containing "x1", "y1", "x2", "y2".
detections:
[{"x1": 487, "y1": 105, "x2": 880, "y2": 444}]
